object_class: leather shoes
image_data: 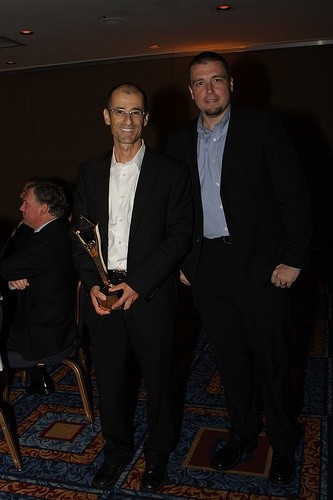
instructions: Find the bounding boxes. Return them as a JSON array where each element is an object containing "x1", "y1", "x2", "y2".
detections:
[
  {"x1": 269, "y1": 426, "x2": 303, "y2": 485},
  {"x1": 25, "y1": 371, "x2": 53, "y2": 395},
  {"x1": 93, "y1": 446, "x2": 134, "y2": 488},
  {"x1": 209, "y1": 432, "x2": 258, "y2": 470},
  {"x1": 141, "y1": 459, "x2": 168, "y2": 492}
]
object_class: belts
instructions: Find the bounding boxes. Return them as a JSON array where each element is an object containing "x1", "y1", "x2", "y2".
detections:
[
  {"x1": 107, "y1": 270, "x2": 129, "y2": 281},
  {"x1": 215, "y1": 236, "x2": 232, "y2": 245}
]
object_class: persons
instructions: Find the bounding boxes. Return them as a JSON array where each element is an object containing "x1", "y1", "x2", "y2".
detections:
[
  {"x1": 6, "y1": 177, "x2": 49, "y2": 245},
  {"x1": 0, "y1": 182, "x2": 79, "y2": 396},
  {"x1": 68, "y1": 82, "x2": 193, "y2": 493},
  {"x1": 165, "y1": 51, "x2": 313, "y2": 486}
]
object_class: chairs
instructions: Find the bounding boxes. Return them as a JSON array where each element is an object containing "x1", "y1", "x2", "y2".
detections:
[{"x1": 0, "y1": 281, "x2": 95, "y2": 472}]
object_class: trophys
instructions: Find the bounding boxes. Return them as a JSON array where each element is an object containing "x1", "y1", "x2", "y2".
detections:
[{"x1": 72, "y1": 218, "x2": 123, "y2": 310}]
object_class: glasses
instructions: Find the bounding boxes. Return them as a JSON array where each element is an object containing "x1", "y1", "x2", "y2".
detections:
[{"x1": 107, "y1": 107, "x2": 147, "y2": 120}]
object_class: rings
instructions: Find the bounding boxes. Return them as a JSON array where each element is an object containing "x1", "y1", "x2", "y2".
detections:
[{"x1": 280, "y1": 282, "x2": 287, "y2": 285}]
